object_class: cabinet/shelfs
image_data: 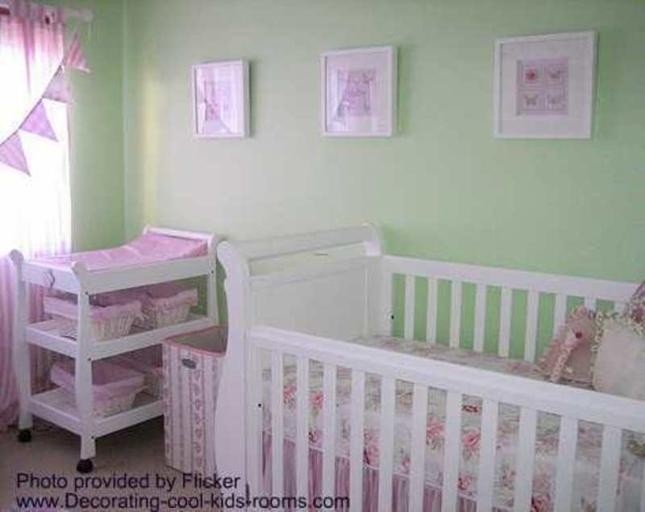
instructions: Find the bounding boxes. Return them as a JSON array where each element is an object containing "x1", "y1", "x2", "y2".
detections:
[{"x1": 13, "y1": 223, "x2": 219, "y2": 471}]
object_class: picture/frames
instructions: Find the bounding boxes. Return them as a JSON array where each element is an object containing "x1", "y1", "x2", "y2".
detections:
[
  {"x1": 319, "y1": 45, "x2": 398, "y2": 141},
  {"x1": 188, "y1": 59, "x2": 251, "y2": 140},
  {"x1": 493, "y1": 29, "x2": 599, "y2": 142}
]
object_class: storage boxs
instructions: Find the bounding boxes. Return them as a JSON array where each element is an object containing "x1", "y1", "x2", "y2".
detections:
[
  {"x1": 163, "y1": 318, "x2": 225, "y2": 483},
  {"x1": 42, "y1": 282, "x2": 198, "y2": 418}
]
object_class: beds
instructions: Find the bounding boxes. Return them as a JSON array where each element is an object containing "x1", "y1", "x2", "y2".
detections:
[{"x1": 213, "y1": 221, "x2": 645, "y2": 512}]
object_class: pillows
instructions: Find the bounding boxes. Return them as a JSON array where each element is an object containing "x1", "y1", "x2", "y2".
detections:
[
  {"x1": 621, "y1": 279, "x2": 645, "y2": 331},
  {"x1": 591, "y1": 308, "x2": 645, "y2": 458}
]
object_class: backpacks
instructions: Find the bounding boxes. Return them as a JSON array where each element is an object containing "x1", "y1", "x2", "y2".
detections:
[{"x1": 96, "y1": 284, "x2": 197, "y2": 330}]
